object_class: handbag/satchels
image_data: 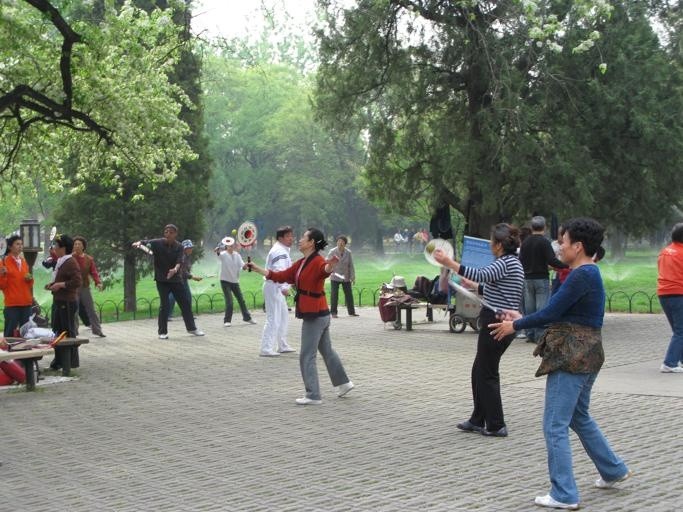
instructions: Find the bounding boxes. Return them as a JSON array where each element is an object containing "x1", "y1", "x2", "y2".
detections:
[{"x1": 0, "y1": 337, "x2": 42, "y2": 351}]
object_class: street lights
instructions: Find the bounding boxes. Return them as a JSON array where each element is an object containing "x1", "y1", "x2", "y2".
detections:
[{"x1": 18, "y1": 217, "x2": 43, "y2": 300}]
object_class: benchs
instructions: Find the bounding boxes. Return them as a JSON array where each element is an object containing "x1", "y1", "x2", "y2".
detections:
[
  {"x1": 396, "y1": 302, "x2": 455, "y2": 331},
  {"x1": 0, "y1": 351, "x2": 44, "y2": 392},
  {"x1": 40, "y1": 335, "x2": 89, "y2": 376}
]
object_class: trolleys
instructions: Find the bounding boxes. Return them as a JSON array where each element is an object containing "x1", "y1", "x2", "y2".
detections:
[{"x1": 448, "y1": 289, "x2": 482, "y2": 334}]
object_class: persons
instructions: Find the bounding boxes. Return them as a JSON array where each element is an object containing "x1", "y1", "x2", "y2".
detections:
[
  {"x1": 394, "y1": 227, "x2": 428, "y2": 255},
  {"x1": 256, "y1": 226, "x2": 297, "y2": 356},
  {"x1": 167, "y1": 238, "x2": 203, "y2": 322},
  {"x1": 0, "y1": 234, "x2": 106, "y2": 373},
  {"x1": 130, "y1": 223, "x2": 205, "y2": 339},
  {"x1": 325, "y1": 236, "x2": 360, "y2": 318},
  {"x1": 656, "y1": 220, "x2": 683, "y2": 374},
  {"x1": 215, "y1": 241, "x2": 257, "y2": 327},
  {"x1": 428, "y1": 211, "x2": 605, "y2": 436},
  {"x1": 487, "y1": 214, "x2": 633, "y2": 511},
  {"x1": 242, "y1": 228, "x2": 354, "y2": 405}
]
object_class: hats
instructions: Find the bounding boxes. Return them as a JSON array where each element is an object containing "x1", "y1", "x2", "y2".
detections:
[{"x1": 182, "y1": 240, "x2": 194, "y2": 249}]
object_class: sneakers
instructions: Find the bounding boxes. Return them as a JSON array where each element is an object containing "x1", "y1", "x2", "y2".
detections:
[
  {"x1": 332, "y1": 314, "x2": 359, "y2": 319},
  {"x1": 295, "y1": 396, "x2": 322, "y2": 404},
  {"x1": 186, "y1": 329, "x2": 205, "y2": 336},
  {"x1": 260, "y1": 346, "x2": 296, "y2": 356},
  {"x1": 659, "y1": 362, "x2": 683, "y2": 372},
  {"x1": 248, "y1": 318, "x2": 257, "y2": 325},
  {"x1": 224, "y1": 322, "x2": 232, "y2": 327},
  {"x1": 44, "y1": 366, "x2": 76, "y2": 372},
  {"x1": 596, "y1": 470, "x2": 633, "y2": 489},
  {"x1": 534, "y1": 494, "x2": 579, "y2": 510},
  {"x1": 160, "y1": 331, "x2": 169, "y2": 340},
  {"x1": 337, "y1": 381, "x2": 354, "y2": 396},
  {"x1": 457, "y1": 420, "x2": 508, "y2": 436}
]
